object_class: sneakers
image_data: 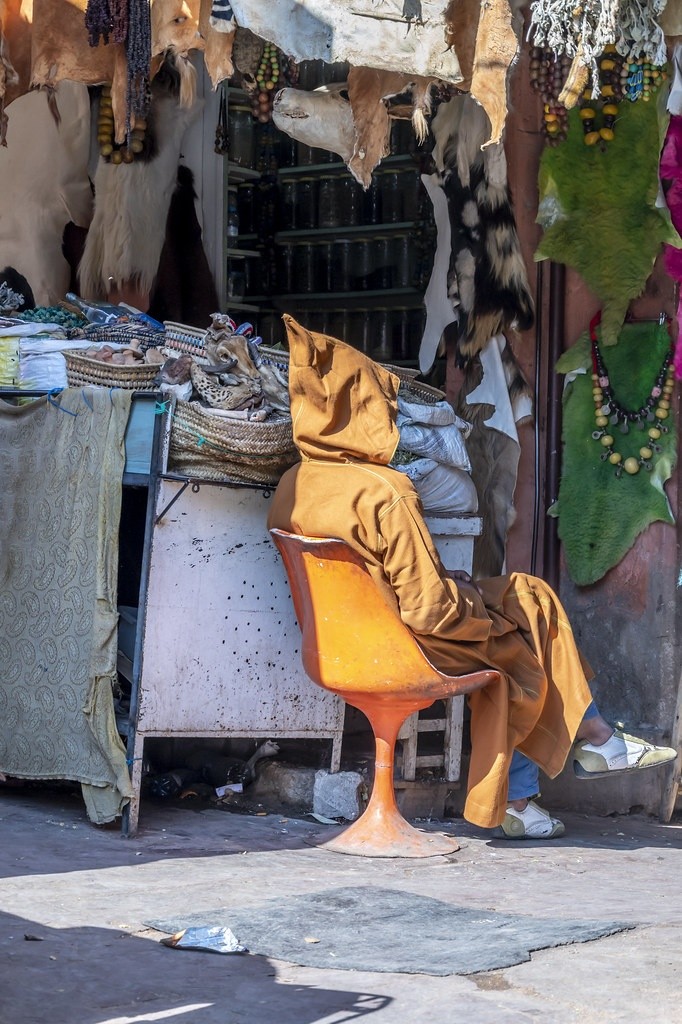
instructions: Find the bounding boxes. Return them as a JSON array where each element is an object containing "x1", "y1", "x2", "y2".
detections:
[
  {"x1": 491, "y1": 793, "x2": 565, "y2": 839},
  {"x1": 573, "y1": 722, "x2": 678, "y2": 780}
]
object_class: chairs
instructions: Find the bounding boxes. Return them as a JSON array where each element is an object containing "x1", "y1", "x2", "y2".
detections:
[{"x1": 269, "y1": 527, "x2": 497, "y2": 857}]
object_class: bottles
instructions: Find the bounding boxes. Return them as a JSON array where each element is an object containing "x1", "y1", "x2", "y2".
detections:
[
  {"x1": 299, "y1": 59, "x2": 351, "y2": 92},
  {"x1": 186, "y1": 750, "x2": 252, "y2": 788},
  {"x1": 228, "y1": 106, "x2": 437, "y2": 362},
  {"x1": 66, "y1": 293, "x2": 132, "y2": 324}
]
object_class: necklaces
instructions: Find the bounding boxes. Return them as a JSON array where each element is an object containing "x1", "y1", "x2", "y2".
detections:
[
  {"x1": 251, "y1": 41, "x2": 300, "y2": 123},
  {"x1": 589, "y1": 310, "x2": 675, "y2": 478},
  {"x1": 529, "y1": 0, "x2": 668, "y2": 147},
  {"x1": 98, "y1": 88, "x2": 146, "y2": 164}
]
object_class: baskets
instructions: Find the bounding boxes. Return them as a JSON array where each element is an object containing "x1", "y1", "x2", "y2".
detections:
[
  {"x1": 375, "y1": 363, "x2": 446, "y2": 404},
  {"x1": 167, "y1": 398, "x2": 301, "y2": 486},
  {"x1": 59, "y1": 348, "x2": 164, "y2": 393},
  {"x1": 162, "y1": 320, "x2": 290, "y2": 377},
  {"x1": 83, "y1": 322, "x2": 168, "y2": 350}
]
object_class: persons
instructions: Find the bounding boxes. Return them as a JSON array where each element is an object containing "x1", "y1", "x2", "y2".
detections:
[{"x1": 268, "y1": 312, "x2": 679, "y2": 839}]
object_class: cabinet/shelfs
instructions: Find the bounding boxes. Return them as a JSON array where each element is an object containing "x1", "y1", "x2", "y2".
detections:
[{"x1": 185, "y1": 71, "x2": 436, "y2": 366}]
